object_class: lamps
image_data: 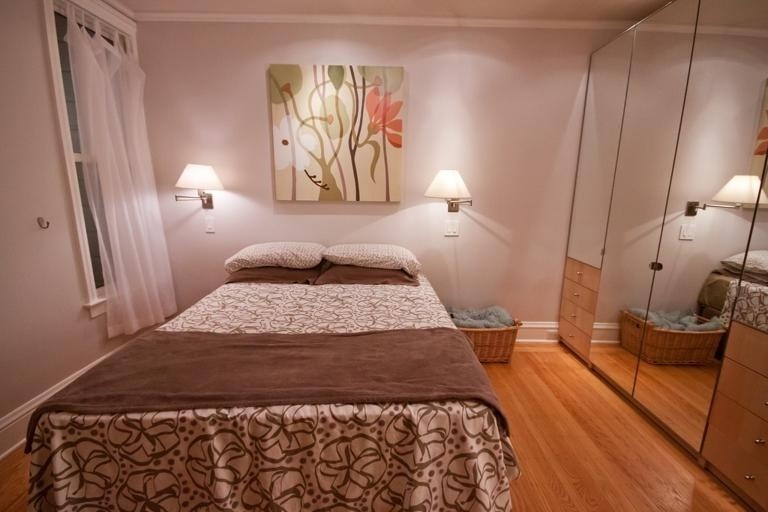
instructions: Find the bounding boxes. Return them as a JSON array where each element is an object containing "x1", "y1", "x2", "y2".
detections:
[
  {"x1": 175, "y1": 164, "x2": 223, "y2": 209},
  {"x1": 423, "y1": 169, "x2": 472, "y2": 213}
]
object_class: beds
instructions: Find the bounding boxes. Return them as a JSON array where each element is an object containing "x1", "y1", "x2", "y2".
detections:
[{"x1": 25, "y1": 271, "x2": 522, "y2": 511}]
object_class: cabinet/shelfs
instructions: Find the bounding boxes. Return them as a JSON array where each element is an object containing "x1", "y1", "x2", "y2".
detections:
[
  {"x1": 565, "y1": 0, "x2": 768, "y2": 460},
  {"x1": 697, "y1": 321, "x2": 768, "y2": 512},
  {"x1": 557, "y1": 256, "x2": 601, "y2": 369}
]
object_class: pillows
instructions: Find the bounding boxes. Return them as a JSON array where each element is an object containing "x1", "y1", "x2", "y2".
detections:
[
  {"x1": 315, "y1": 264, "x2": 420, "y2": 286},
  {"x1": 223, "y1": 259, "x2": 331, "y2": 285},
  {"x1": 224, "y1": 242, "x2": 326, "y2": 274},
  {"x1": 321, "y1": 244, "x2": 423, "y2": 277}
]
